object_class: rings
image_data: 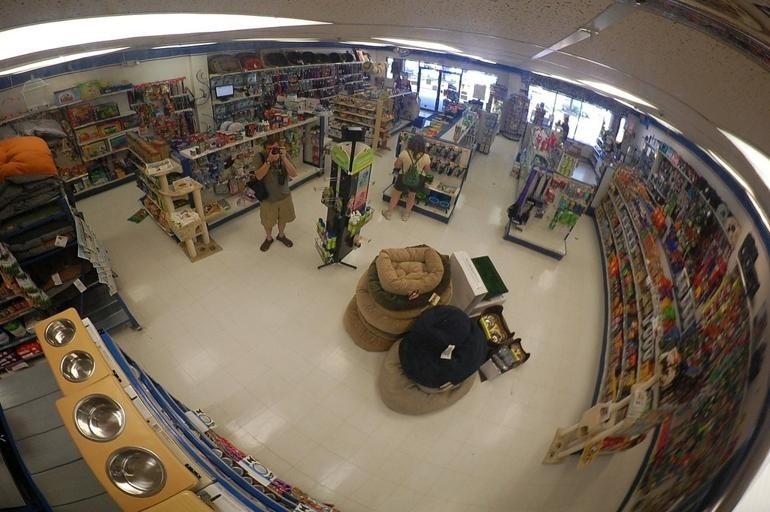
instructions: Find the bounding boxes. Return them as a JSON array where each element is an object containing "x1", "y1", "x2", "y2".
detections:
[{"x1": 277, "y1": 156, "x2": 279, "y2": 158}]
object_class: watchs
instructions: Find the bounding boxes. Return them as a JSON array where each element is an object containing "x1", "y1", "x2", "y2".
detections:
[{"x1": 265, "y1": 158, "x2": 273, "y2": 166}]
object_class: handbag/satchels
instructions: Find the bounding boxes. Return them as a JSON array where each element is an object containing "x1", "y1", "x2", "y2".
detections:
[{"x1": 247, "y1": 179, "x2": 268, "y2": 201}]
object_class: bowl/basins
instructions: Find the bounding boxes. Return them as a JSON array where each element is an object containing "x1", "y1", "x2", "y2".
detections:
[
  {"x1": 105, "y1": 445, "x2": 168, "y2": 497},
  {"x1": 43, "y1": 318, "x2": 77, "y2": 348},
  {"x1": 59, "y1": 350, "x2": 96, "y2": 383},
  {"x1": 71, "y1": 393, "x2": 127, "y2": 443}
]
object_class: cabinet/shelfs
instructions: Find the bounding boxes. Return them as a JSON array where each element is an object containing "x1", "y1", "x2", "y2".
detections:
[
  {"x1": 385, "y1": 98, "x2": 500, "y2": 222},
  {"x1": 506, "y1": 124, "x2": 607, "y2": 260},
  {"x1": 2, "y1": 168, "x2": 143, "y2": 378},
  {"x1": 2, "y1": 78, "x2": 194, "y2": 203}
]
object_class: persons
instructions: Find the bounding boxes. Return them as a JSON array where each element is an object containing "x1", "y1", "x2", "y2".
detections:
[
  {"x1": 561, "y1": 118, "x2": 570, "y2": 143},
  {"x1": 382, "y1": 134, "x2": 430, "y2": 221},
  {"x1": 248, "y1": 137, "x2": 298, "y2": 252}
]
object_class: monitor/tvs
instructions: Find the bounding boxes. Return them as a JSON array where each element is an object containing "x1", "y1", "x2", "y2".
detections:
[{"x1": 215, "y1": 84, "x2": 235, "y2": 101}]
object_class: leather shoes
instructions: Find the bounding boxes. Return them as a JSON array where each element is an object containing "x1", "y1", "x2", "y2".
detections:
[
  {"x1": 276, "y1": 233, "x2": 293, "y2": 247},
  {"x1": 260, "y1": 238, "x2": 274, "y2": 251}
]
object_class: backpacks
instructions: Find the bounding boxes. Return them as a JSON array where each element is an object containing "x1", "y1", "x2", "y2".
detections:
[{"x1": 403, "y1": 150, "x2": 426, "y2": 185}]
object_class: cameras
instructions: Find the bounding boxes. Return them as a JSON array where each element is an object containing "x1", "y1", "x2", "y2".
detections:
[{"x1": 271, "y1": 147, "x2": 282, "y2": 155}]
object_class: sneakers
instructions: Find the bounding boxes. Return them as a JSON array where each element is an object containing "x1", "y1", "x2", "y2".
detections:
[
  {"x1": 401, "y1": 208, "x2": 411, "y2": 221},
  {"x1": 382, "y1": 209, "x2": 391, "y2": 220}
]
